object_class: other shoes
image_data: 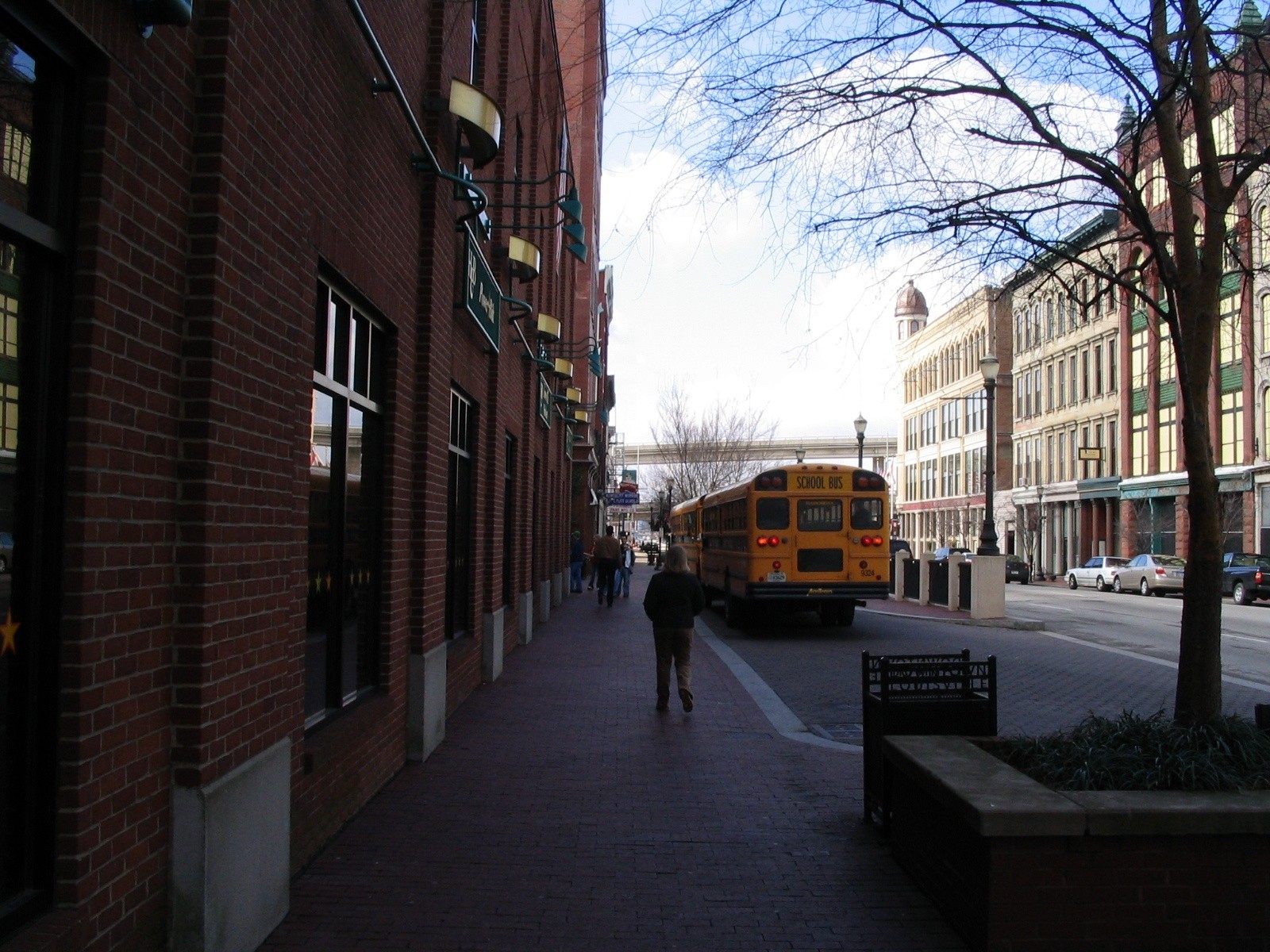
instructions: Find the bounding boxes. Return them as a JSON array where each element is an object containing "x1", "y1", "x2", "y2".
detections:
[
  {"x1": 678, "y1": 688, "x2": 693, "y2": 713},
  {"x1": 587, "y1": 585, "x2": 594, "y2": 591},
  {"x1": 655, "y1": 692, "x2": 669, "y2": 711}
]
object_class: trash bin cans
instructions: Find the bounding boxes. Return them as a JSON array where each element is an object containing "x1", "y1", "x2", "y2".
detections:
[{"x1": 862, "y1": 645, "x2": 1000, "y2": 832}]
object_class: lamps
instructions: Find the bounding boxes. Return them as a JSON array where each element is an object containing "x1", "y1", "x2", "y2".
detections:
[
  {"x1": 491, "y1": 235, "x2": 542, "y2": 283},
  {"x1": 568, "y1": 410, "x2": 588, "y2": 424},
  {"x1": 442, "y1": 76, "x2": 504, "y2": 169},
  {"x1": 546, "y1": 337, "x2": 603, "y2": 378},
  {"x1": 459, "y1": 162, "x2": 588, "y2": 265},
  {"x1": 581, "y1": 401, "x2": 608, "y2": 427},
  {"x1": 526, "y1": 312, "x2": 561, "y2": 345},
  {"x1": 547, "y1": 358, "x2": 574, "y2": 380},
  {"x1": 558, "y1": 387, "x2": 581, "y2": 405}
]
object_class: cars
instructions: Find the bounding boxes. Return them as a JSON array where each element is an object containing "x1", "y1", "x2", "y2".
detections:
[
  {"x1": 1112, "y1": 553, "x2": 1187, "y2": 597},
  {"x1": 963, "y1": 552, "x2": 977, "y2": 563},
  {"x1": 1064, "y1": 556, "x2": 1131, "y2": 593},
  {"x1": 889, "y1": 539, "x2": 913, "y2": 559},
  {"x1": 933, "y1": 547, "x2": 971, "y2": 559},
  {"x1": 645, "y1": 542, "x2": 658, "y2": 553},
  {"x1": 1000, "y1": 554, "x2": 1029, "y2": 586}
]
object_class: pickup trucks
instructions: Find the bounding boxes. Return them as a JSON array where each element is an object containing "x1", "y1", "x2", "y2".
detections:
[{"x1": 1222, "y1": 552, "x2": 1270, "y2": 606}]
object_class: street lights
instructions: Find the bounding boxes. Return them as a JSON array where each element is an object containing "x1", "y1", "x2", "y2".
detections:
[
  {"x1": 854, "y1": 412, "x2": 867, "y2": 468},
  {"x1": 978, "y1": 348, "x2": 1000, "y2": 555},
  {"x1": 649, "y1": 500, "x2": 654, "y2": 565},
  {"x1": 667, "y1": 477, "x2": 675, "y2": 514},
  {"x1": 658, "y1": 490, "x2": 665, "y2": 566}
]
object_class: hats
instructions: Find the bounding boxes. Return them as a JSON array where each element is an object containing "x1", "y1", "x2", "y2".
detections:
[{"x1": 572, "y1": 530, "x2": 581, "y2": 537}]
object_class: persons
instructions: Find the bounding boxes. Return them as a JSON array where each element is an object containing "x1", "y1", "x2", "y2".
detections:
[
  {"x1": 614, "y1": 534, "x2": 636, "y2": 598},
  {"x1": 643, "y1": 546, "x2": 706, "y2": 713},
  {"x1": 852, "y1": 500, "x2": 878, "y2": 529},
  {"x1": 569, "y1": 530, "x2": 584, "y2": 593},
  {"x1": 583, "y1": 533, "x2": 605, "y2": 591},
  {"x1": 593, "y1": 525, "x2": 623, "y2": 608}
]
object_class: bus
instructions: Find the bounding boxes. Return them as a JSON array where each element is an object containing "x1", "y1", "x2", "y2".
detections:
[
  {"x1": 697, "y1": 463, "x2": 891, "y2": 631},
  {"x1": 668, "y1": 494, "x2": 706, "y2": 579}
]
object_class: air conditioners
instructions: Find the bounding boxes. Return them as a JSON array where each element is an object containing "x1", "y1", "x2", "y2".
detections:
[{"x1": 1019, "y1": 477, "x2": 1029, "y2": 486}]
